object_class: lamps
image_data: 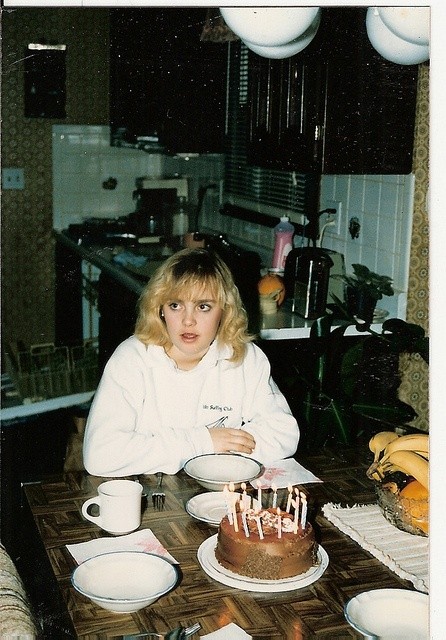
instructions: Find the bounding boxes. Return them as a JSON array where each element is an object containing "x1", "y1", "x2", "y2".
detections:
[
  {"x1": 366, "y1": 8, "x2": 430, "y2": 66},
  {"x1": 218, "y1": 7, "x2": 321, "y2": 44},
  {"x1": 240, "y1": 11, "x2": 321, "y2": 60},
  {"x1": 376, "y1": 6, "x2": 429, "y2": 46}
]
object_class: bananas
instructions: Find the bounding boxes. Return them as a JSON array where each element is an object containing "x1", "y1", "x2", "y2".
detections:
[
  {"x1": 375, "y1": 432, "x2": 429, "y2": 461},
  {"x1": 368, "y1": 430, "x2": 399, "y2": 462},
  {"x1": 381, "y1": 450, "x2": 429, "y2": 486}
]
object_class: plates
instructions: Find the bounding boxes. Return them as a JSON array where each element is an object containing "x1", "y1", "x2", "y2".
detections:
[
  {"x1": 344, "y1": 588, "x2": 429, "y2": 639},
  {"x1": 197, "y1": 532, "x2": 329, "y2": 592}
]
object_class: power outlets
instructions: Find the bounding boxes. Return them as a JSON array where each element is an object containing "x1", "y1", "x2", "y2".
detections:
[
  {"x1": 206, "y1": 179, "x2": 222, "y2": 197},
  {"x1": 2, "y1": 168, "x2": 23, "y2": 190},
  {"x1": 326, "y1": 200, "x2": 341, "y2": 235}
]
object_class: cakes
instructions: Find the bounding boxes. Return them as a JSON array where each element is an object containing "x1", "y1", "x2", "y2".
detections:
[{"x1": 215, "y1": 479, "x2": 316, "y2": 578}]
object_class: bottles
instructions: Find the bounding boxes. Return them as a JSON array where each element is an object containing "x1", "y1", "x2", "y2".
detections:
[{"x1": 172, "y1": 196, "x2": 189, "y2": 237}]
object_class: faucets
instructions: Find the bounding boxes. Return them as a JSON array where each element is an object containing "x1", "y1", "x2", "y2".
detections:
[{"x1": 193, "y1": 231, "x2": 240, "y2": 257}]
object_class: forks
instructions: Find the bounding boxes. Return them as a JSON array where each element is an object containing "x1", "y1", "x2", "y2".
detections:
[{"x1": 152, "y1": 472, "x2": 166, "y2": 513}]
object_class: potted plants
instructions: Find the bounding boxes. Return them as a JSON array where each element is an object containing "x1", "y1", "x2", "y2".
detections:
[{"x1": 332, "y1": 264, "x2": 395, "y2": 327}]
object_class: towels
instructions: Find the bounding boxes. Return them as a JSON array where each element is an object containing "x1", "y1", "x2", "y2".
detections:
[
  {"x1": 250, "y1": 458, "x2": 324, "y2": 490},
  {"x1": 65, "y1": 528, "x2": 180, "y2": 565}
]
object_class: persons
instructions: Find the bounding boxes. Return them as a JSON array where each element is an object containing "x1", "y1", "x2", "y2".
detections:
[{"x1": 82, "y1": 246, "x2": 301, "y2": 477}]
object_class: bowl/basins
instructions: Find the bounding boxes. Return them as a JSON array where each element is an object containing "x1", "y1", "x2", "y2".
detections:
[
  {"x1": 374, "y1": 476, "x2": 430, "y2": 539},
  {"x1": 185, "y1": 491, "x2": 262, "y2": 528},
  {"x1": 184, "y1": 454, "x2": 266, "y2": 490},
  {"x1": 72, "y1": 551, "x2": 179, "y2": 614}
]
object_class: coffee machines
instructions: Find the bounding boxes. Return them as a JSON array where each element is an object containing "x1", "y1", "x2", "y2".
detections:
[{"x1": 131, "y1": 176, "x2": 194, "y2": 243}]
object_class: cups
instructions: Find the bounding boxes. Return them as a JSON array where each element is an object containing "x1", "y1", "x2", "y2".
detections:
[
  {"x1": 259, "y1": 295, "x2": 281, "y2": 315},
  {"x1": 82, "y1": 480, "x2": 144, "y2": 537}
]
